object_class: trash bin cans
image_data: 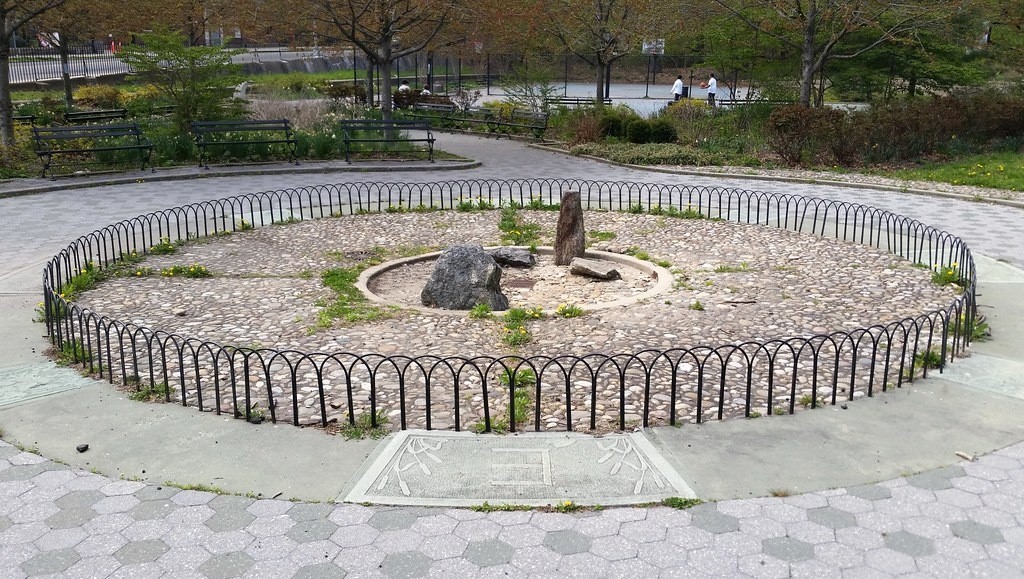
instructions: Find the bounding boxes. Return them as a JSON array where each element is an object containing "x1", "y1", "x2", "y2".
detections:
[{"x1": 681, "y1": 87, "x2": 689, "y2": 98}]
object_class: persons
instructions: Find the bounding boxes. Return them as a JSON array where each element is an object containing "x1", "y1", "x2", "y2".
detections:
[
  {"x1": 669, "y1": 75, "x2": 683, "y2": 101},
  {"x1": 700, "y1": 73, "x2": 716, "y2": 106},
  {"x1": 398, "y1": 80, "x2": 411, "y2": 98},
  {"x1": 420, "y1": 84, "x2": 432, "y2": 95}
]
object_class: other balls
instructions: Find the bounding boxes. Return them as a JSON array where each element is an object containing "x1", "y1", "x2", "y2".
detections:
[{"x1": 701, "y1": 82, "x2": 706, "y2": 87}]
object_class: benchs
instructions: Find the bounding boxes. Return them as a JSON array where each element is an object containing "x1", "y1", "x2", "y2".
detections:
[
  {"x1": 448, "y1": 104, "x2": 504, "y2": 138},
  {"x1": 191, "y1": 119, "x2": 300, "y2": 170},
  {"x1": 339, "y1": 119, "x2": 437, "y2": 164},
  {"x1": 13, "y1": 114, "x2": 37, "y2": 129},
  {"x1": 543, "y1": 98, "x2": 611, "y2": 111},
  {"x1": 51, "y1": 108, "x2": 128, "y2": 133},
  {"x1": 494, "y1": 109, "x2": 549, "y2": 144},
  {"x1": 136, "y1": 106, "x2": 197, "y2": 129},
  {"x1": 404, "y1": 103, "x2": 459, "y2": 134},
  {"x1": 712, "y1": 99, "x2": 810, "y2": 114},
  {"x1": 31, "y1": 123, "x2": 156, "y2": 181}
]
